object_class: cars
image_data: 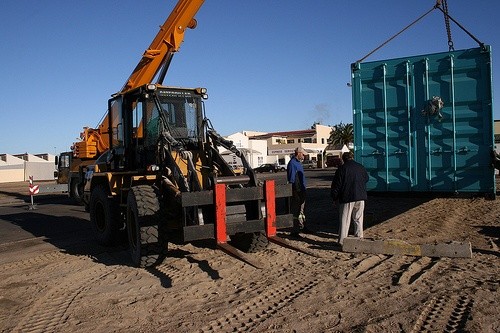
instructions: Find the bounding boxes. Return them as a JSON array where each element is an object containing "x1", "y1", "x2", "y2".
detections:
[{"x1": 230, "y1": 164, "x2": 242, "y2": 176}]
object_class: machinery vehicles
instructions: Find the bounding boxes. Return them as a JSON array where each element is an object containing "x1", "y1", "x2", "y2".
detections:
[
  {"x1": 89, "y1": 83, "x2": 293, "y2": 270},
  {"x1": 56, "y1": 0, "x2": 204, "y2": 204}
]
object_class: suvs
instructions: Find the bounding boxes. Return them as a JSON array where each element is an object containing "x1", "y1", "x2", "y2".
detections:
[{"x1": 302, "y1": 160, "x2": 317, "y2": 169}]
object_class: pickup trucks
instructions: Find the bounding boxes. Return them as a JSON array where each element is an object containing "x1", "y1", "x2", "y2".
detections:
[
  {"x1": 276, "y1": 164, "x2": 287, "y2": 172},
  {"x1": 253, "y1": 163, "x2": 281, "y2": 173}
]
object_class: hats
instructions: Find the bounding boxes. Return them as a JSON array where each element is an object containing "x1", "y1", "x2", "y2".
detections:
[{"x1": 296, "y1": 146, "x2": 307, "y2": 155}]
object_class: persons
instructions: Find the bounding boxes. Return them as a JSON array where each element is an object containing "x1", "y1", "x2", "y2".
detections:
[
  {"x1": 287, "y1": 146, "x2": 314, "y2": 238},
  {"x1": 331, "y1": 152, "x2": 369, "y2": 246},
  {"x1": 147, "y1": 110, "x2": 170, "y2": 144}
]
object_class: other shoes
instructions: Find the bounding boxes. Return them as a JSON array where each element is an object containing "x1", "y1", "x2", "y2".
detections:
[{"x1": 300, "y1": 227, "x2": 311, "y2": 234}]
